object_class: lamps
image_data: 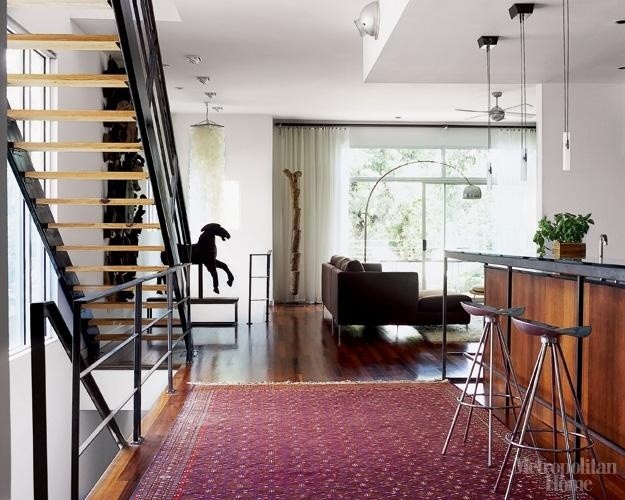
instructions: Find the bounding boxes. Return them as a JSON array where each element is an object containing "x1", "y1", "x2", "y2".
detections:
[
  {"x1": 365, "y1": 162, "x2": 482, "y2": 264},
  {"x1": 564, "y1": 0, "x2": 570, "y2": 171},
  {"x1": 477, "y1": 2, "x2": 533, "y2": 190}
]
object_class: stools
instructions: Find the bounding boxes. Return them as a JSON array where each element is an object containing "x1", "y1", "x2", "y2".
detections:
[{"x1": 442, "y1": 300, "x2": 608, "y2": 500}]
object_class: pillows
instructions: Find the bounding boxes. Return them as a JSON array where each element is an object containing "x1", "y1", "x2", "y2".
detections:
[{"x1": 330, "y1": 255, "x2": 365, "y2": 272}]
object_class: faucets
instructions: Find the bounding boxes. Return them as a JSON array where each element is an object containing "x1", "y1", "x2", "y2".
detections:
[{"x1": 599, "y1": 233, "x2": 609, "y2": 263}]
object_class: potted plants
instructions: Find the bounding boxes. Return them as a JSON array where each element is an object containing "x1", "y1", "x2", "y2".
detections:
[{"x1": 534, "y1": 213, "x2": 595, "y2": 261}]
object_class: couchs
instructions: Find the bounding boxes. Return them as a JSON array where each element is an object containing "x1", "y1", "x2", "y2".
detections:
[{"x1": 322, "y1": 255, "x2": 472, "y2": 349}]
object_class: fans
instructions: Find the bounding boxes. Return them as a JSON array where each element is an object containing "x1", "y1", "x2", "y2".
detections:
[{"x1": 454, "y1": 91, "x2": 536, "y2": 124}]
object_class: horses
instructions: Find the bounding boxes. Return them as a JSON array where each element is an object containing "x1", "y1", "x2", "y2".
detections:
[{"x1": 156, "y1": 223, "x2": 234, "y2": 296}]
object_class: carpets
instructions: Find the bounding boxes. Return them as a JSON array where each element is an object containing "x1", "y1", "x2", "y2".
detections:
[
  {"x1": 120, "y1": 380, "x2": 605, "y2": 499},
  {"x1": 414, "y1": 314, "x2": 485, "y2": 343}
]
